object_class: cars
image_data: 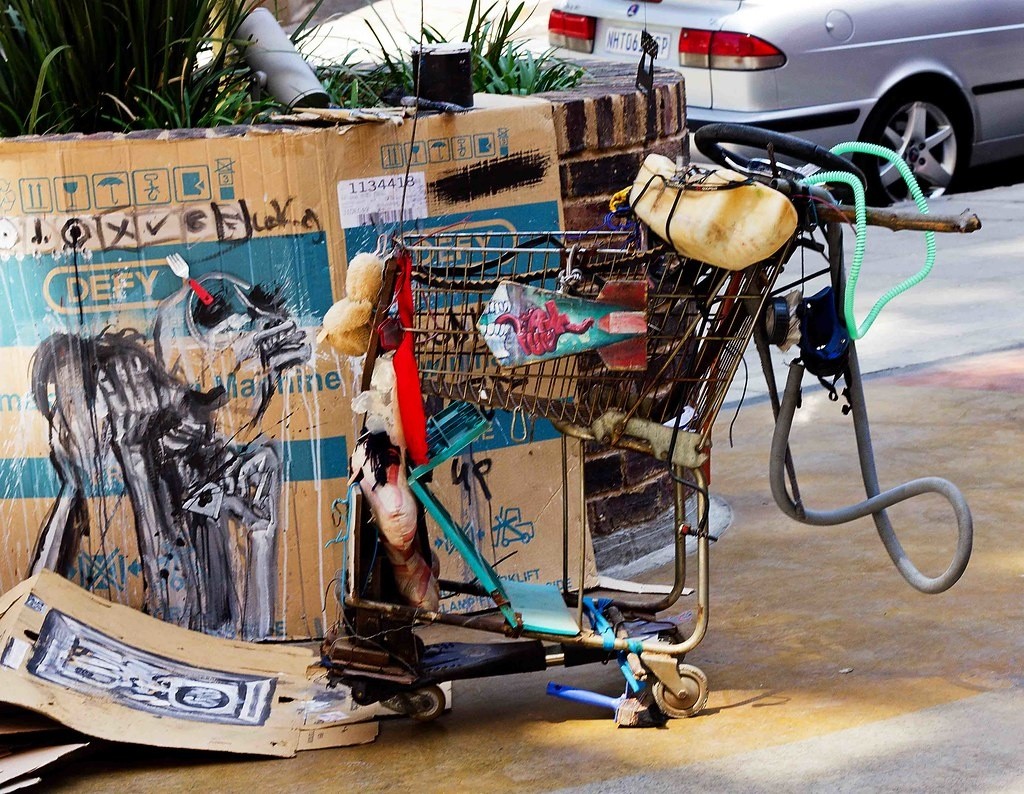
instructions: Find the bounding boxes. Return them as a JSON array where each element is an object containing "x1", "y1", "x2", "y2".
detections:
[{"x1": 546, "y1": 0, "x2": 1024, "y2": 206}]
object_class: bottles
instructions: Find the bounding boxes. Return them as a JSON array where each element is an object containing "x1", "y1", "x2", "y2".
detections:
[
  {"x1": 229, "y1": 6, "x2": 333, "y2": 115},
  {"x1": 410, "y1": 42, "x2": 475, "y2": 111}
]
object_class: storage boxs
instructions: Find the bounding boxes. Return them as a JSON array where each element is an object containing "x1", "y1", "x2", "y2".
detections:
[{"x1": 1, "y1": 92, "x2": 604, "y2": 647}]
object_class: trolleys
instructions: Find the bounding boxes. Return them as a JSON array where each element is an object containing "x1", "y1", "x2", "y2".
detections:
[{"x1": 318, "y1": 153, "x2": 883, "y2": 728}]
